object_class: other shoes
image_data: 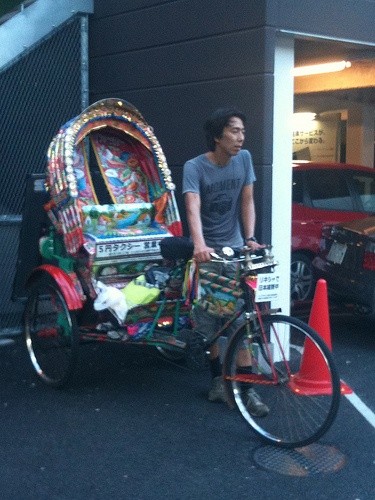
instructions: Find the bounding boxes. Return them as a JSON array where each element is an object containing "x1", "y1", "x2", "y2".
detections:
[
  {"x1": 208, "y1": 374, "x2": 230, "y2": 402},
  {"x1": 242, "y1": 387, "x2": 270, "y2": 416}
]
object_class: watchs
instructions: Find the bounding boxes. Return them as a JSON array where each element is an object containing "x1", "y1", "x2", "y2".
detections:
[{"x1": 245, "y1": 237, "x2": 257, "y2": 243}]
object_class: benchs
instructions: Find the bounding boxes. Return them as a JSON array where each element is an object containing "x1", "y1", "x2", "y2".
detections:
[{"x1": 74, "y1": 203, "x2": 174, "y2": 284}]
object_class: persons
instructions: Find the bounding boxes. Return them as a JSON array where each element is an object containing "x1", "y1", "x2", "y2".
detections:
[{"x1": 181, "y1": 108, "x2": 270, "y2": 418}]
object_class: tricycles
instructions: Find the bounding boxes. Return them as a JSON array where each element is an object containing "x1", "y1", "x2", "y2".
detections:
[{"x1": 19, "y1": 97, "x2": 342, "y2": 449}]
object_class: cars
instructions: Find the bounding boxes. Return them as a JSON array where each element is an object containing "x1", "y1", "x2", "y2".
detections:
[
  {"x1": 291, "y1": 162, "x2": 375, "y2": 297},
  {"x1": 310, "y1": 219, "x2": 375, "y2": 321}
]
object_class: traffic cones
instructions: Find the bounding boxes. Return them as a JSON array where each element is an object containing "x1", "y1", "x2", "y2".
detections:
[{"x1": 290, "y1": 278, "x2": 349, "y2": 399}]
